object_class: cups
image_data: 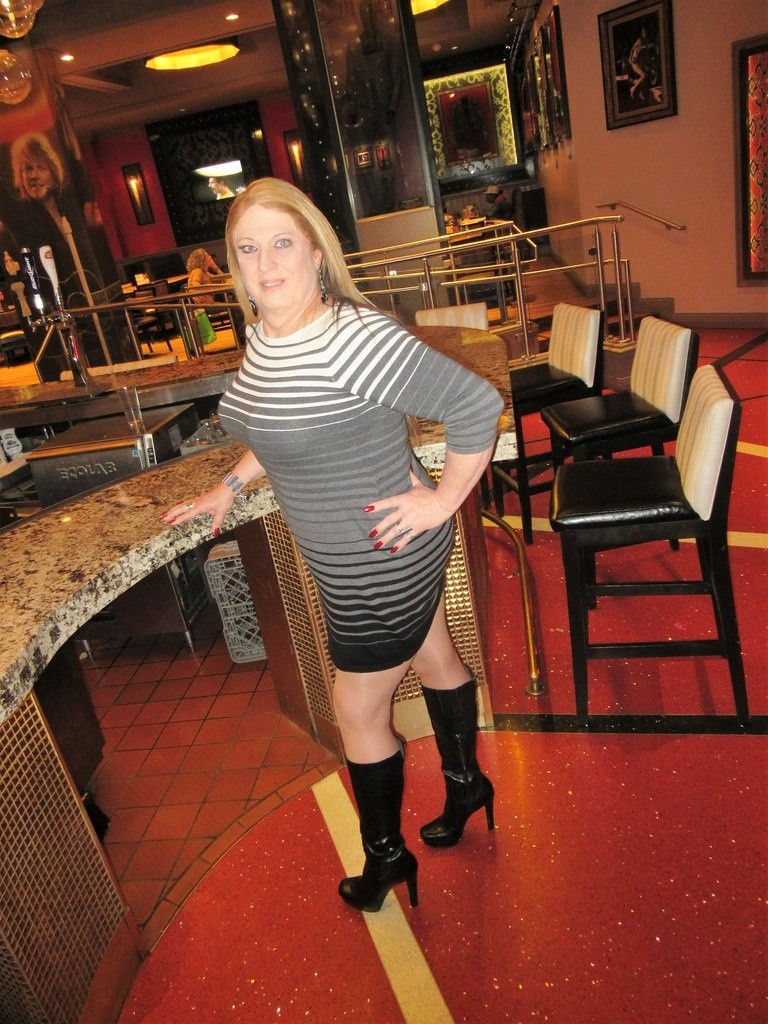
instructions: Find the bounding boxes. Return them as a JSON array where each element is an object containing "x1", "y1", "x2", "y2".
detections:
[
  {"x1": 181, "y1": 407, "x2": 232, "y2": 448},
  {"x1": 118, "y1": 386, "x2": 144, "y2": 427}
]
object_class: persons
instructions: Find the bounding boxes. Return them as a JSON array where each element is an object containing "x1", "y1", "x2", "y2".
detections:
[
  {"x1": 186, "y1": 248, "x2": 243, "y2": 323},
  {"x1": 157, "y1": 177, "x2": 503, "y2": 912},
  {"x1": 2, "y1": 132, "x2": 118, "y2": 357},
  {"x1": 628, "y1": 26, "x2": 650, "y2": 100},
  {"x1": 207, "y1": 175, "x2": 235, "y2": 201},
  {"x1": 473, "y1": 185, "x2": 512, "y2": 223}
]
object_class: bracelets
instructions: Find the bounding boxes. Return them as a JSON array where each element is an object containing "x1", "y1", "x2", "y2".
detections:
[
  {"x1": 213, "y1": 267, "x2": 220, "y2": 271},
  {"x1": 221, "y1": 472, "x2": 244, "y2": 495}
]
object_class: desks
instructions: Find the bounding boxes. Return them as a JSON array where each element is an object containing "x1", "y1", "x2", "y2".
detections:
[
  {"x1": 445, "y1": 209, "x2": 516, "y2": 292},
  {"x1": 1, "y1": 316, "x2": 523, "y2": 1024}
]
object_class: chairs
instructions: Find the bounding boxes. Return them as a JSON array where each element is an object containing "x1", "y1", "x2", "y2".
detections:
[
  {"x1": 551, "y1": 363, "x2": 753, "y2": 718},
  {"x1": 119, "y1": 250, "x2": 241, "y2": 352},
  {"x1": 540, "y1": 313, "x2": 702, "y2": 552},
  {"x1": 489, "y1": 302, "x2": 609, "y2": 545}
]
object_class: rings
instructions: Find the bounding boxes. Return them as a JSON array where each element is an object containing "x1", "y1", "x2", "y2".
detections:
[
  {"x1": 406, "y1": 532, "x2": 415, "y2": 540},
  {"x1": 397, "y1": 522, "x2": 404, "y2": 534},
  {"x1": 185, "y1": 502, "x2": 193, "y2": 510}
]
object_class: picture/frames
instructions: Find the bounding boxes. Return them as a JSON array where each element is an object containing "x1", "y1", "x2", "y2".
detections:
[
  {"x1": 729, "y1": 32, "x2": 767, "y2": 290},
  {"x1": 594, "y1": 1, "x2": 679, "y2": 131},
  {"x1": 436, "y1": 82, "x2": 500, "y2": 166}
]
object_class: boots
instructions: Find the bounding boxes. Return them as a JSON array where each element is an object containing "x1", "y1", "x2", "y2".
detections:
[
  {"x1": 336, "y1": 733, "x2": 420, "y2": 916},
  {"x1": 419, "y1": 660, "x2": 495, "y2": 850}
]
